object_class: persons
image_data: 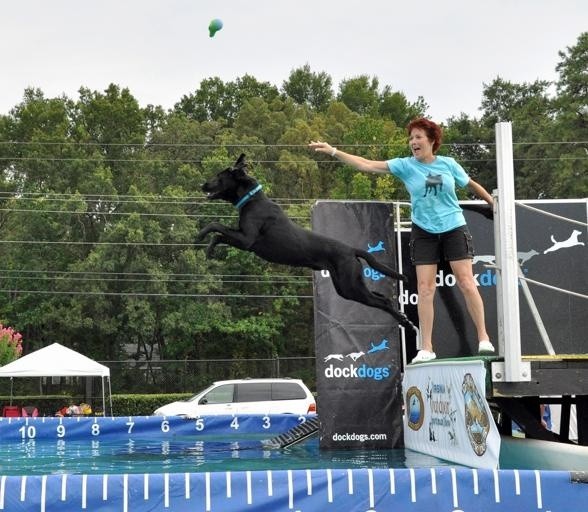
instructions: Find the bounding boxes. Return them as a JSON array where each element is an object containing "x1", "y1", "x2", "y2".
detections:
[
  {"x1": 307, "y1": 119, "x2": 500, "y2": 362},
  {"x1": 62, "y1": 399, "x2": 94, "y2": 416}
]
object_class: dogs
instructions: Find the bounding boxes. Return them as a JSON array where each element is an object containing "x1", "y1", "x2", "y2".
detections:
[{"x1": 194, "y1": 152, "x2": 414, "y2": 331}]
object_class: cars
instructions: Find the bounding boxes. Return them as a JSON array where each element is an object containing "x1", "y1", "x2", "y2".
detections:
[{"x1": 151, "y1": 377, "x2": 316, "y2": 416}]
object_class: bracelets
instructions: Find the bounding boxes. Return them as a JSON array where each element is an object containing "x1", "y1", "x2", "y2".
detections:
[{"x1": 329, "y1": 146, "x2": 339, "y2": 159}]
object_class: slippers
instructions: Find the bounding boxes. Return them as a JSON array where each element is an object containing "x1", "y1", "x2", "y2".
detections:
[
  {"x1": 478, "y1": 341, "x2": 495, "y2": 353},
  {"x1": 413, "y1": 350, "x2": 436, "y2": 363}
]
